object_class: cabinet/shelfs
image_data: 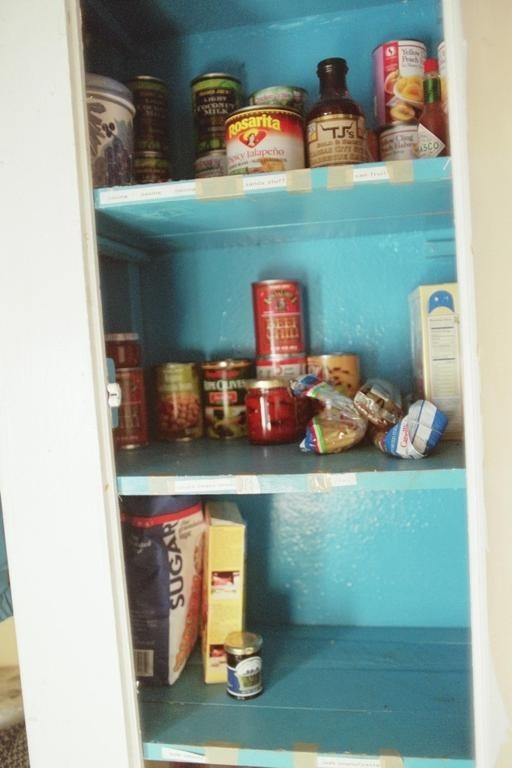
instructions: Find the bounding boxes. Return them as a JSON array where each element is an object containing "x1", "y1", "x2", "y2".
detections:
[{"x1": 66, "y1": 0, "x2": 507, "y2": 765}]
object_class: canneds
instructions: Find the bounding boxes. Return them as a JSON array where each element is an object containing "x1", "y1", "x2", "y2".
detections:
[
  {"x1": 223, "y1": 630, "x2": 263, "y2": 701},
  {"x1": 105, "y1": 279, "x2": 358, "y2": 450},
  {"x1": 127, "y1": 38, "x2": 445, "y2": 183}
]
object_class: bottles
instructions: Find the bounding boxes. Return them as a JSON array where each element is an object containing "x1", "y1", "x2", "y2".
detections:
[
  {"x1": 304, "y1": 57, "x2": 368, "y2": 168},
  {"x1": 244, "y1": 378, "x2": 298, "y2": 446},
  {"x1": 416, "y1": 56, "x2": 450, "y2": 159}
]
